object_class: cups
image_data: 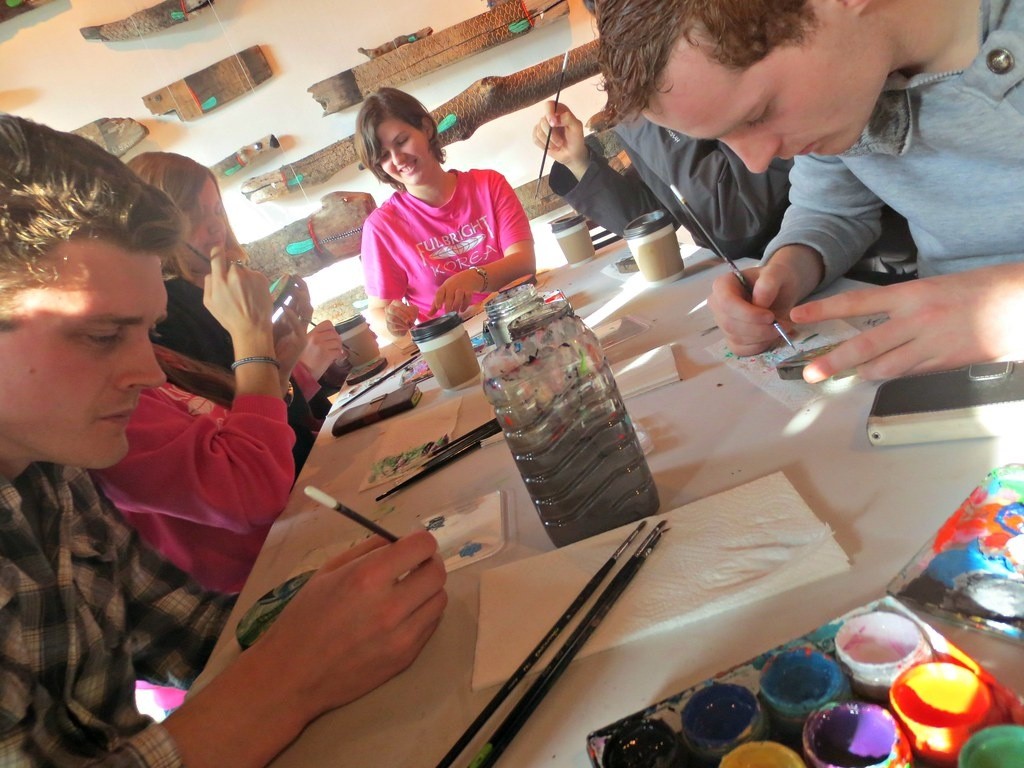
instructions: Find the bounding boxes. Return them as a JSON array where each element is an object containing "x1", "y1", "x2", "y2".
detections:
[
  {"x1": 334, "y1": 316, "x2": 381, "y2": 369},
  {"x1": 622, "y1": 210, "x2": 688, "y2": 286},
  {"x1": 410, "y1": 310, "x2": 482, "y2": 392},
  {"x1": 551, "y1": 214, "x2": 596, "y2": 268}
]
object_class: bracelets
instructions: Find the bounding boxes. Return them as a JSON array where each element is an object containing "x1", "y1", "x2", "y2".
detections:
[{"x1": 231, "y1": 356, "x2": 282, "y2": 371}]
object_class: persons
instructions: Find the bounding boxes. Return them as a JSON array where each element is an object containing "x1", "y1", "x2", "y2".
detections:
[
  {"x1": 590, "y1": 0, "x2": 1024, "y2": 385},
  {"x1": 355, "y1": 87, "x2": 537, "y2": 337},
  {"x1": 292, "y1": 320, "x2": 343, "y2": 403},
  {"x1": 1, "y1": 114, "x2": 449, "y2": 768},
  {"x1": 126, "y1": 151, "x2": 312, "y2": 483},
  {"x1": 532, "y1": 0, "x2": 916, "y2": 276},
  {"x1": 84, "y1": 248, "x2": 297, "y2": 718}
]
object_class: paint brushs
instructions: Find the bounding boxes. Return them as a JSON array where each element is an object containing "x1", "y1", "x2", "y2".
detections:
[
  {"x1": 669, "y1": 182, "x2": 801, "y2": 356},
  {"x1": 182, "y1": 237, "x2": 211, "y2": 268},
  {"x1": 435, "y1": 516, "x2": 671, "y2": 768},
  {"x1": 324, "y1": 353, "x2": 420, "y2": 418},
  {"x1": 270, "y1": 290, "x2": 362, "y2": 359},
  {"x1": 302, "y1": 483, "x2": 400, "y2": 544},
  {"x1": 532, "y1": 49, "x2": 571, "y2": 203},
  {"x1": 375, "y1": 417, "x2": 502, "y2": 503}
]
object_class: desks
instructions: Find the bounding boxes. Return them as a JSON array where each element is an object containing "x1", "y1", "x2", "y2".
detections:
[{"x1": 176, "y1": 218, "x2": 1023, "y2": 767}]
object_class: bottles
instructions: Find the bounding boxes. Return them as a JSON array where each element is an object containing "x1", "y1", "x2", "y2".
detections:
[
  {"x1": 480, "y1": 316, "x2": 659, "y2": 550},
  {"x1": 507, "y1": 302, "x2": 585, "y2": 344},
  {"x1": 485, "y1": 283, "x2": 543, "y2": 348},
  {"x1": 601, "y1": 612, "x2": 1024, "y2": 768}
]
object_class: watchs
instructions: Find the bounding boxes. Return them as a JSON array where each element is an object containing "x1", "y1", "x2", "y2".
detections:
[{"x1": 469, "y1": 265, "x2": 488, "y2": 292}]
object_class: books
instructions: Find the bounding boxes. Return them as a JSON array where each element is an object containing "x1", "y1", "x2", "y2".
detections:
[
  {"x1": 331, "y1": 381, "x2": 423, "y2": 437},
  {"x1": 866, "y1": 360, "x2": 1024, "y2": 446}
]
